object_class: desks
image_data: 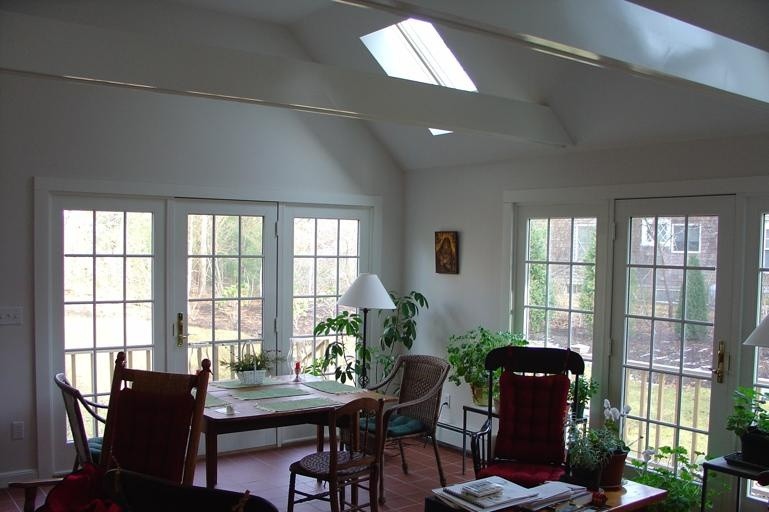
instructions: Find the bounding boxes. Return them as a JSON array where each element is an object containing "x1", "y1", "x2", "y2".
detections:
[
  {"x1": 424, "y1": 479, "x2": 667, "y2": 512},
  {"x1": 191, "y1": 373, "x2": 398, "y2": 488}
]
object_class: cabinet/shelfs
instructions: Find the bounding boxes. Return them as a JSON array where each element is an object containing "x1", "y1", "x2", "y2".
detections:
[{"x1": 699, "y1": 456, "x2": 769, "y2": 512}]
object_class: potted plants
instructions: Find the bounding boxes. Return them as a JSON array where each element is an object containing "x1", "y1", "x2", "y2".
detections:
[
  {"x1": 567, "y1": 424, "x2": 605, "y2": 490},
  {"x1": 599, "y1": 399, "x2": 630, "y2": 490},
  {"x1": 725, "y1": 386, "x2": 769, "y2": 465},
  {"x1": 220, "y1": 340, "x2": 287, "y2": 384},
  {"x1": 569, "y1": 376, "x2": 599, "y2": 419},
  {"x1": 447, "y1": 325, "x2": 528, "y2": 406}
]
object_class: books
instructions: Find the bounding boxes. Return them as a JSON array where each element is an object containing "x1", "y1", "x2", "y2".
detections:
[{"x1": 432, "y1": 474, "x2": 592, "y2": 512}]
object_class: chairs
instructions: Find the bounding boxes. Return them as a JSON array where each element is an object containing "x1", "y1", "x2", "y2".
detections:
[
  {"x1": 101, "y1": 468, "x2": 278, "y2": 512},
  {"x1": 287, "y1": 398, "x2": 385, "y2": 512},
  {"x1": 53, "y1": 372, "x2": 109, "y2": 475},
  {"x1": 8, "y1": 351, "x2": 210, "y2": 512},
  {"x1": 471, "y1": 346, "x2": 585, "y2": 488},
  {"x1": 340, "y1": 353, "x2": 450, "y2": 487}
]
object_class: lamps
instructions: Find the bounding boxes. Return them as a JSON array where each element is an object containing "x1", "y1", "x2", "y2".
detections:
[
  {"x1": 335, "y1": 274, "x2": 397, "y2": 386},
  {"x1": 742, "y1": 315, "x2": 768, "y2": 347}
]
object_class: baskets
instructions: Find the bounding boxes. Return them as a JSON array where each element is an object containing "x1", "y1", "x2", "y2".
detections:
[{"x1": 236, "y1": 340, "x2": 267, "y2": 385}]
